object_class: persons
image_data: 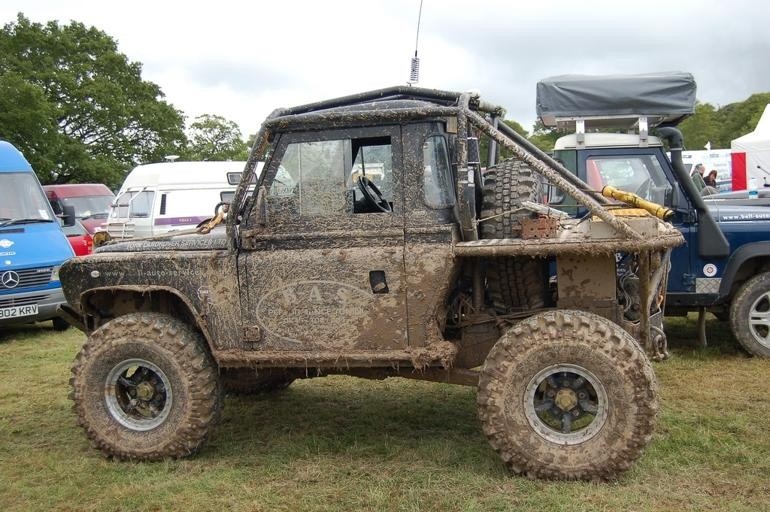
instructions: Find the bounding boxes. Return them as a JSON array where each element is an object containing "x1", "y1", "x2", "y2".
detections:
[
  {"x1": 691, "y1": 164, "x2": 704, "y2": 178},
  {"x1": 702, "y1": 170, "x2": 718, "y2": 188}
]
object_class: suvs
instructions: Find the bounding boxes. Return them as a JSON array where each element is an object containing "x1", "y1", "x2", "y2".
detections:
[{"x1": 56, "y1": 1, "x2": 687, "y2": 482}]
